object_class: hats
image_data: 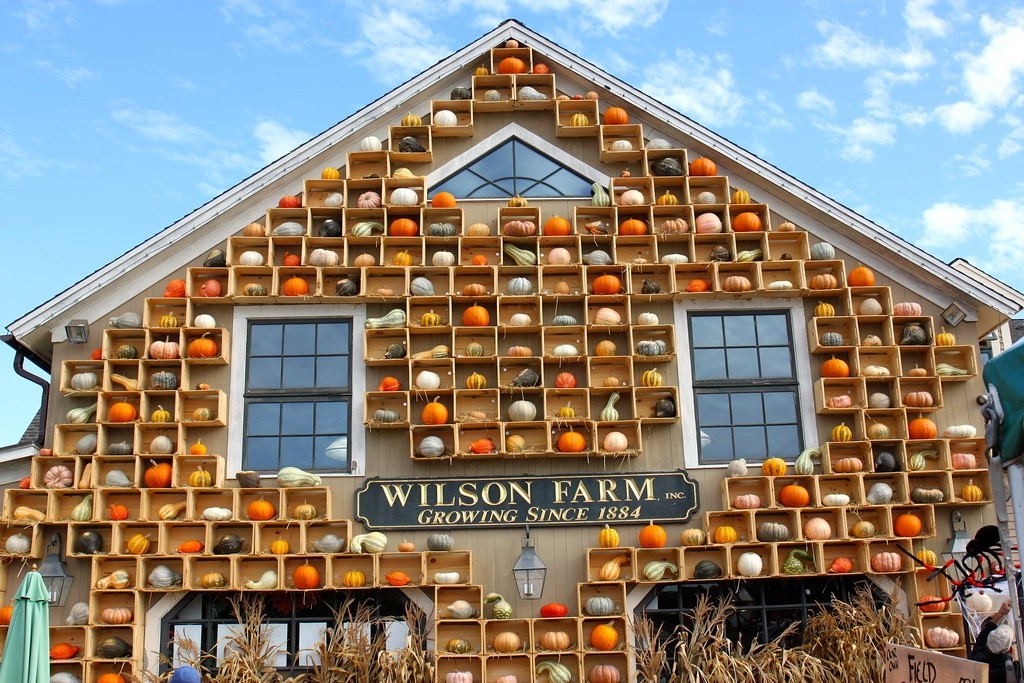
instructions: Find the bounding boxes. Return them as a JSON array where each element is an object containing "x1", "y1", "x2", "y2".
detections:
[
  {"x1": 988, "y1": 625, "x2": 1014, "y2": 655},
  {"x1": 168, "y1": 666, "x2": 200, "y2": 683}
]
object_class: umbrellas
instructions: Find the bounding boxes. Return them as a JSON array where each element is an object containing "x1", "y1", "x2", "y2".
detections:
[{"x1": 0, "y1": 563, "x2": 52, "y2": 683}]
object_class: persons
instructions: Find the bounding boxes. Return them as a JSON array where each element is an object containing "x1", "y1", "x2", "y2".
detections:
[{"x1": 969, "y1": 600, "x2": 1022, "y2": 683}]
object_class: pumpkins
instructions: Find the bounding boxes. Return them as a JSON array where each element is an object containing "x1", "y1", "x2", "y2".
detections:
[{"x1": 0, "y1": 38, "x2": 992, "y2": 683}]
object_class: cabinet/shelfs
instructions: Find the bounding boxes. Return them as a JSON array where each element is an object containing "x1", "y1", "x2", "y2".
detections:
[{"x1": 0, "y1": 47, "x2": 995, "y2": 683}]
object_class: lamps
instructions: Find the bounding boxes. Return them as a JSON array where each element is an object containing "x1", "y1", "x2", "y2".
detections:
[
  {"x1": 512, "y1": 525, "x2": 548, "y2": 601},
  {"x1": 36, "y1": 531, "x2": 74, "y2": 608},
  {"x1": 939, "y1": 301, "x2": 967, "y2": 328},
  {"x1": 940, "y1": 507, "x2": 974, "y2": 584},
  {"x1": 64, "y1": 319, "x2": 91, "y2": 344}
]
